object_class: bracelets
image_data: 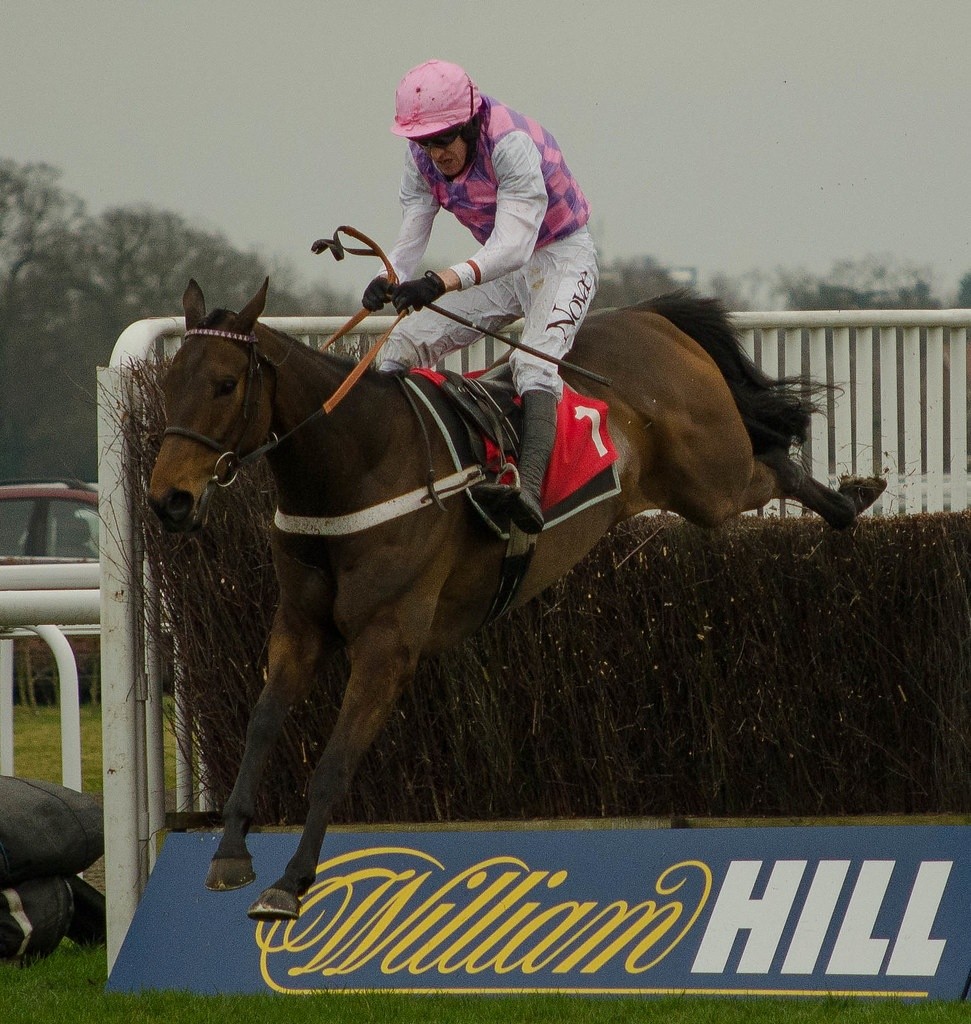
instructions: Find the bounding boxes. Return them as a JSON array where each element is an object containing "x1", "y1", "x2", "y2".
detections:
[{"x1": 467, "y1": 257, "x2": 482, "y2": 286}]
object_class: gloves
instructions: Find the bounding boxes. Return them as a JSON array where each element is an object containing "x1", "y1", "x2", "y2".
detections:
[
  {"x1": 362, "y1": 275, "x2": 392, "y2": 312},
  {"x1": 391, "y1": 270, "x2": 446, "y2": 316}
]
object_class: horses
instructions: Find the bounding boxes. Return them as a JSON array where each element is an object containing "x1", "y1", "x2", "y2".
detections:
[{"x1": 144, "y1": 273, "x2": 893, "y2": 923}]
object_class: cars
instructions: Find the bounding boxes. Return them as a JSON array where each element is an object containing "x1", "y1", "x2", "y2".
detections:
[{"x1": 0, "y1": 479, "x2": 175, "y2": 704}]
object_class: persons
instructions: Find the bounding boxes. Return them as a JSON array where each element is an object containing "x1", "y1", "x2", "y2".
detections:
[{"x1": 361, "y1": 59, "x2": 601, "y2": 535}]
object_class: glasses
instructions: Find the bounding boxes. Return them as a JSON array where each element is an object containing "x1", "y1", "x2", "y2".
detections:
[{"x1": 406, "y1": 124, "x2": 465, "y2": 148}]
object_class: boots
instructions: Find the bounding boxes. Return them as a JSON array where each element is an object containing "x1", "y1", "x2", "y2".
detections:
[{"x1": 472, "y1": 389, "x2": 558, "y2": 534}]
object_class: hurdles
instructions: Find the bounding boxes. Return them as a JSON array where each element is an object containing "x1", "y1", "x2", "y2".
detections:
[{"x1": 104, "y1": 819, "x2": 971, "y2": 1004}]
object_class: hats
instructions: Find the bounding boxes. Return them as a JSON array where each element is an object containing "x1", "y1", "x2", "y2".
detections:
[{"x1": 391, "y1": 59, "x2": 483, "y2": 137}]
